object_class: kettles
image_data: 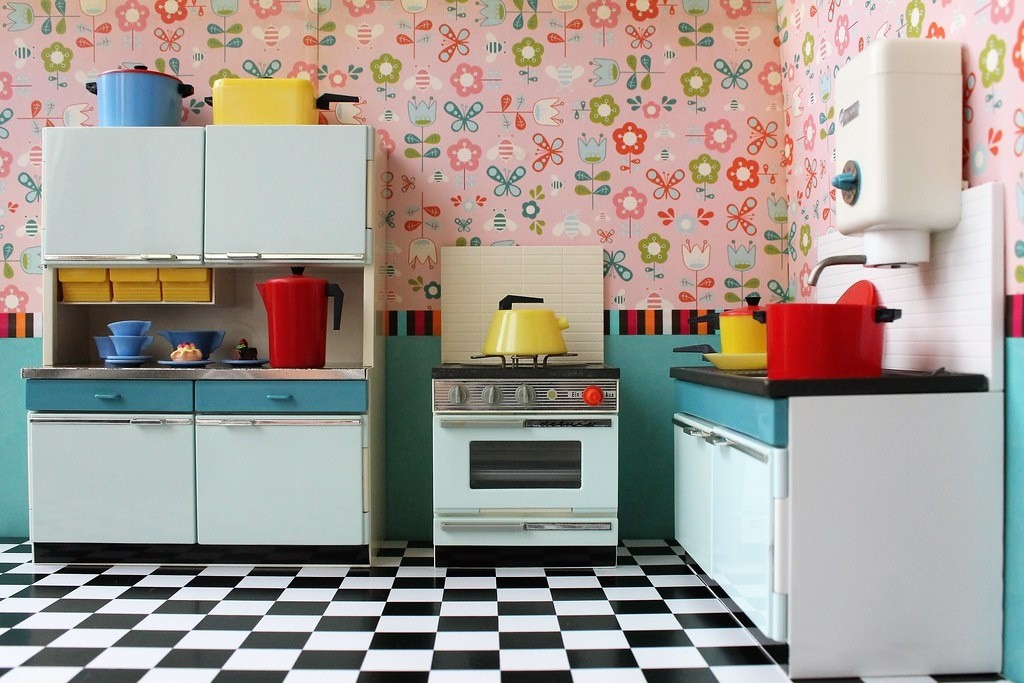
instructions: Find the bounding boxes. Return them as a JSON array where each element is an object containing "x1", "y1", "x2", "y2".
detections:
[{"x1": 480, "y1": 293, "x2": 569, "y2": 357}]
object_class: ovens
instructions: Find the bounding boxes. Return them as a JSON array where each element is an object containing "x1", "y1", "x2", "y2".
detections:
[{"x1": 431, "y1": 415, "x2": 618, "y2": 568}]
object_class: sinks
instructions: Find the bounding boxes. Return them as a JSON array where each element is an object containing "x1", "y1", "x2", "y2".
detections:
[{"x1": 720, "y1": 370, "x2": 950, "y2": 380}]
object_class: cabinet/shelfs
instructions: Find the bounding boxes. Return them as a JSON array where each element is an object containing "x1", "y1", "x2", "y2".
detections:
[
  {"x1": 193, "y1": 380, "x2": 387, "y2": 567},
  {"x1": 670, "y1": 380, "x2": 1007, "y2": 681},
  {"x1": 203, "y1": 125, "x2": 390, "y2": 268},
  {"x1": 41, "y1": 126, "x2": 203, "y2": 268},
  {"x1": 24, "y1": 379, "x2": 195, "y2": 566}
]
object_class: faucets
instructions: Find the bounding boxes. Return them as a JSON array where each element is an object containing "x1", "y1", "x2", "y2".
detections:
[{"x1": 809, "y1": 256, "x2": 866, "y2": 287}]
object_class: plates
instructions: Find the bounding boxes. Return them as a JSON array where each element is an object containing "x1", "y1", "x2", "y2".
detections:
[
  {"x1": 105, "y1": 355, "x2": 154, "y2": 364},
  {"x1": 220, "y1": 360, "x2": 268, "y2": 368},
  {"x1": 158, "y1": 360, "x2": 215, "y2": 368},
  {"x1": 673, "y1": 344, "x2": 767, "y2": 370}
]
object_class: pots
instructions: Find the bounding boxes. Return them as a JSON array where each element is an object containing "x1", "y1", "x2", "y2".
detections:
[
  {"x1": 749, "y1": 301, "x2": 904, "y2": 383},
  {"x1": 84, "y1": 64, "x2": 195, "y2": 127},
  {"x1": 203, "y1": 77, "x2": 360, "y2": 126},
  {"x1": 717, "y1": 296, "x2": 767, "y2": 352}
]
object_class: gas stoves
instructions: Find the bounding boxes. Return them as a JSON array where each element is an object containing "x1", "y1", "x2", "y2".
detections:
[{"x1": 430, "y1": 355, "x2": 622, "y2": 422}]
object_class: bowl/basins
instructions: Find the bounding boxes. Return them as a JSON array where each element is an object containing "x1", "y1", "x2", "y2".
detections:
[
  {"x1": 92, "y1": 320, "x2": 155, "y2": 358},
  {"x1": 156, "y1": 330, "x2": 226, "y2": 359}
]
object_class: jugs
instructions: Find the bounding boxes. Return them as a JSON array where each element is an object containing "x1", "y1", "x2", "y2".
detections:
[{"x1": 255, "y1": 265, "x2": 345, "y2": 369}]
